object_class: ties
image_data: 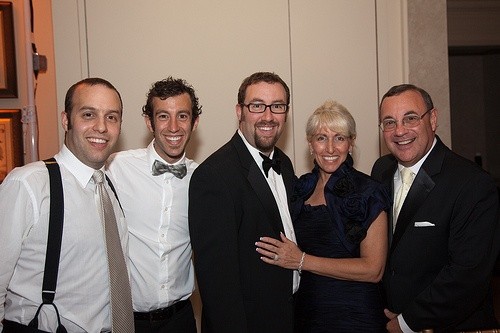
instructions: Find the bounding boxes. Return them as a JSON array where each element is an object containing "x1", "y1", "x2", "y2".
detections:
[
  {"x1": 393, "y1": 169, "x2": 414, "y2": 232},
  {"x1": 92, "y1": 170, "x2": 136, "y2": 333}
]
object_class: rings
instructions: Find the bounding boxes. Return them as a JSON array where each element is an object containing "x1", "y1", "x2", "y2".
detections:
[{"x1": 274, "y1": 254, "x2": 279, "y2": 260}]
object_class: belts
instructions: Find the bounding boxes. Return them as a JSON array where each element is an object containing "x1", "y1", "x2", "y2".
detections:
[{"x1": 134, "y1": 300, "x2": 188, "y2": 322}]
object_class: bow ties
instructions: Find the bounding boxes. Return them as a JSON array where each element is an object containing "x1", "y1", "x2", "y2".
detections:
[
  {"x1": 258, "y1": 151, "x2": 283, "y2": 178},
  {"x1": 151, "y1": 159, "x2": 189, "y2": 181}
]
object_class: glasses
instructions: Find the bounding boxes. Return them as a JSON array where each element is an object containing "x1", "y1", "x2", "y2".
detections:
[
  {"x1": 242, "y1": 102, "x2": 289, "y2": 115},
  {"x1": 378, "y1": 108, "x2": 433, "y2": 131}
]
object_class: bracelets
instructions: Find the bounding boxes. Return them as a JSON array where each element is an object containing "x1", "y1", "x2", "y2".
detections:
[{"x1": 299, "y1": 252, "x2": 305, "y2": 274}]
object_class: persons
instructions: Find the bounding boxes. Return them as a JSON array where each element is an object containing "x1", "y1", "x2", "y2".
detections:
[
  {"x1": 108, "y1": 75, "x2": 203, "y2": 333},
  {"x1": 189, "y1": 73, "x2": 304, "y2": 333},
  {"x1": 0, "y1": 78, "x2": 129, "y2": 333},
  {"x1": 255, "y1": 102, "x2": 388, "y2": 333},
  {"x1": 371, "y1": 84, "x2": 500, "y2": 333}
]
